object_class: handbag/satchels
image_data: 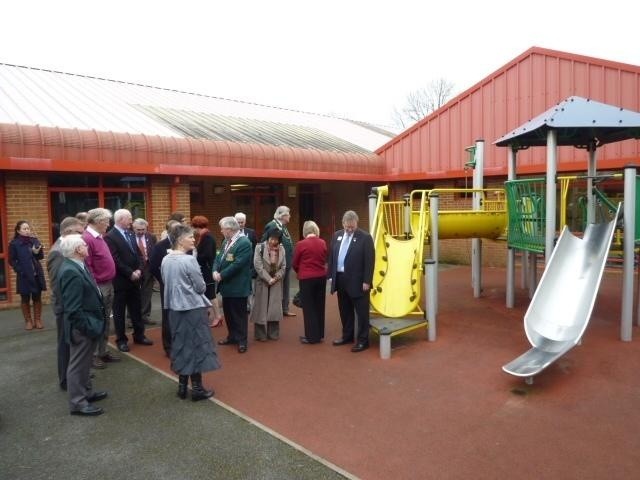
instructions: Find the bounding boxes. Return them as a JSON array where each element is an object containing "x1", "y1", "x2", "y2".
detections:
[
  {"x1": 293, "y1": 290, "x2": 303, "y2": 308},
  {"x1": 250, "y1": 267, "x2": 256, "y2": 278}
]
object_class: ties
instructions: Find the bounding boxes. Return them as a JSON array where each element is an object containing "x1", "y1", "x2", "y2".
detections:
[
  {"x1": 338, "y1": 235, "x2": 349, "y2": 270},
  {"x1": 139, "y1": 237, "x2": 147, "y2": 267},
  {"x1": 84, "y1": 265, "x2": 91, "y2": 278},
  {"x1": 124, "y1": 229, "x2": 134, "y2": 254}
]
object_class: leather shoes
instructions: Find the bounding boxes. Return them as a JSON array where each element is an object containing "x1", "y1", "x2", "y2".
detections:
[
  {"x1": 60, "y1": 313, "x2": 157, "y2": 416},
  {"x1": 205, "y1": 305, "x2": 370, "y2": 354}
]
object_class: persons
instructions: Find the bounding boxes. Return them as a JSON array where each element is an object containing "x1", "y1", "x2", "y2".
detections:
[
  {"x1": 6, "y1": 221, "x2": 49, "y2": 332},
  {"x1": 161, "y1": 225, "x2": 222, "y2": 400},
  {"x1": 77, "y1": 208, "x2": 158, "y2": 369},
  {"x1": 290, "y1": 220, "x2": 327, "y2": 343},
  {"x1": 55, "y1": 234, "x2": 108, "y2": 415},
  {"x1": 327, "y1": 209, "x2": 374, "y2": 351},
  {"x1": 46, "y1": 215, "x2": 95, "y2": 394},
  {"x1": 151, "y1": 207, "x2": 293, "y2": 353}
]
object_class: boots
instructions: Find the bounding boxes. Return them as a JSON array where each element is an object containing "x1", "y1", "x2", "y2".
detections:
[
  {"x1": 34, "y1": 302, "x2": 43, "y2": 329},
  {"x1": 177, "y1": 376, "x2": 187, "y2": 398},
  {"x1": 21, "y1": 303, "x2": 33, "y2": 329},
  {"x1": 191, "y1": 382, "x2": 214, "y2": 400}
]
